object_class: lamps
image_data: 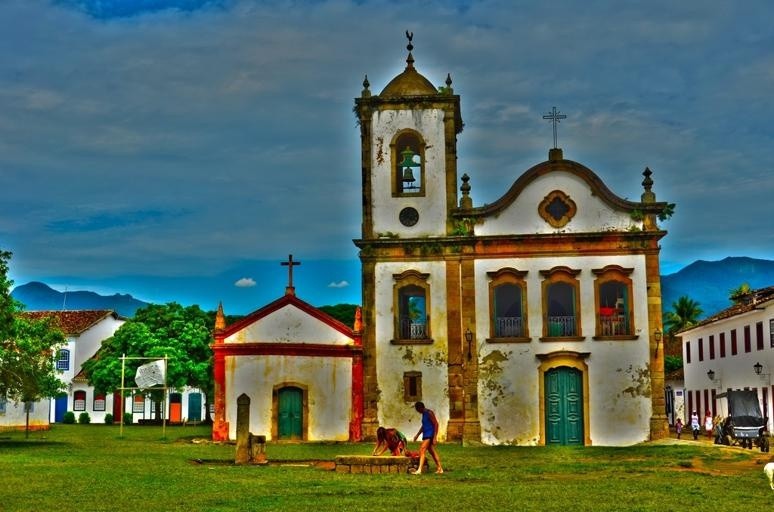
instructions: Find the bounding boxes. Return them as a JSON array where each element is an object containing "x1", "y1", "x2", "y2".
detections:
[
  {"x1": 653, "y1": 327, "x2": 662, "y2": 358},
  {"x1": 753, "y1": 362, "x2": 770, "y2": 375},
  {"x1": 707, "y1": 369, "x2": 720, "y2": 381},
  {"x1": 465, "y1": 327, "x2": 473, "y2": 358}
]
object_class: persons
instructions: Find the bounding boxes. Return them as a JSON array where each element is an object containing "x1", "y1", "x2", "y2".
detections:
[
  {"x1": 687, "y1": 410, "x2": 700, "y2": 439},
  {"x1": 703, "y1": 410, "x2": 713, "y2": 440},
  {"x1": 410, "y1": 401, "x2": 444, "y2": 474},
  {"x1": 372, "y1": 427, "x2": 406, "y2": 455},
  {"x1": 675, "y1": 417, "x2": 684, "y2": 439}
]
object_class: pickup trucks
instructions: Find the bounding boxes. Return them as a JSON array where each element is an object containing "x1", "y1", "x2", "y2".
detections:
[{"x1": 712, "y1": 415, "x2": 766, "y2": 448}]
